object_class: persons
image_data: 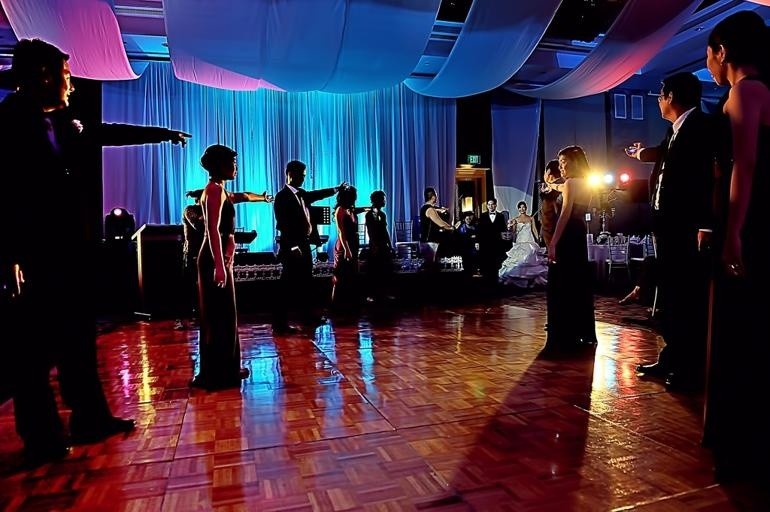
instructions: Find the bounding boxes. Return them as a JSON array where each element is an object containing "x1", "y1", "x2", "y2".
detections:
[
  {"x1": 540, "y1": 159, "x2": 565, "y2": 252},
  {"x1": 270, "y1": 161, "x2": 348, "y2": 336},
  {"x1": 366, "y1": 189, "x2": 396, "y2": 319},
  {"x1": 700, "y1": 10, "x2": 769, "y2": 511},
  {"x1": 1, "y1": 38, "x2": 193, "y2": 458},
  {"x1": 176, "y1": 188, "x2": 204, "y2": 329},
  {"x1": 330, "y1": 185, "x2": 373, "y2": 318},
  {"x1": 457, "y1": 198, "x2": 511, "y2": 305},
  {"x1": 616, "y1": 74, "x2": 720, "y2": 391},
  {"x1": 540, "y1": 144, "x2": 598, "y2": 356},
  {"x1": 498, "y1": 201, "x2": 549, "y2": 290},
  {"x1": 420, "y1": 186, "x2": 461, "y2": 245},
  {"x1": 194, "y1": 144, "x2": 276, "y2": 386}
]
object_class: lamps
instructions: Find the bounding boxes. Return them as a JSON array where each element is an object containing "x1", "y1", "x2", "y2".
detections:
[
  {"x1": 104, "y1": 206, "x2": 137, "y2": 240},
  {"x1": 586, "y1": 170, "x2": 631, "y2": 194}
]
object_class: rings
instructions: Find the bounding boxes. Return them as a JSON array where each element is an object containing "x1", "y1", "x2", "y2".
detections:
[{"x1": 731, "y1": 264, "x2": 736, "y2": 269}]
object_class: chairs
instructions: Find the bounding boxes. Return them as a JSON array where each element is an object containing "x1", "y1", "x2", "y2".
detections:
[
  {"x1": 394, "y1": 220, "x2": 420, "y2": 258},
  {"x1": 358, "y1": 222, "x2": 370, "y2": 251},
  {"x1": 588, "y1": 232, "x2": 658, "y2": 290}
]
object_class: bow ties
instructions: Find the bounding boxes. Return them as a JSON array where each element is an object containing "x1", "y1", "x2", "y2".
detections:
[{"x1": 490, "y1": 213, "x2": 495, "y2": 215}]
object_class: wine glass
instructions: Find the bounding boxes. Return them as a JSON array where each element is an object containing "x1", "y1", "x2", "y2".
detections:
[{"x1": 232, "y1": 255, "x2": 463, "y2": 280}]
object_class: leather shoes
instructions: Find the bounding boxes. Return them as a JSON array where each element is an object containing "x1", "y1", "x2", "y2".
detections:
[
  {"x1": 70, "y1": 414, "x2": 136, "y2": 435},
  {"x1": 637, "y1": 362, "x2": 671, "y2": 375}
]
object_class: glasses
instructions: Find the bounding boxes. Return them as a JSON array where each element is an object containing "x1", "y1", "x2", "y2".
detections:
[{"x1": 658, "y1": 95, "x2": 666, "y2": 102}]
